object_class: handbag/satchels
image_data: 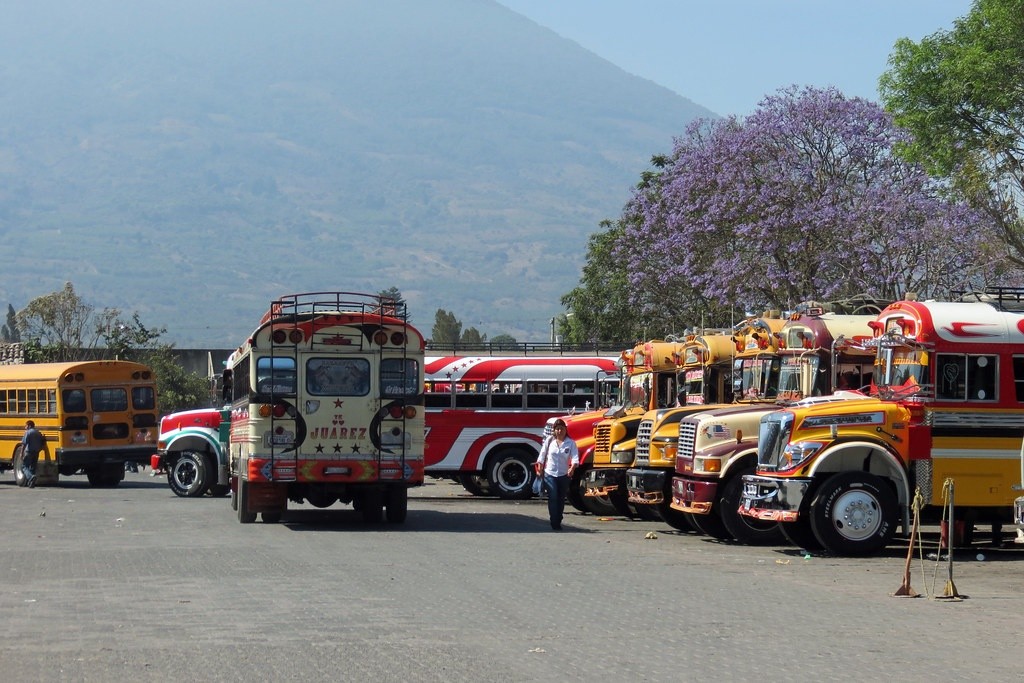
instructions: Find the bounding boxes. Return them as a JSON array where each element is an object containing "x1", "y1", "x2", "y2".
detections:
[{"x1": 537, "y1": 476, "x2": 545, "y2": 497}]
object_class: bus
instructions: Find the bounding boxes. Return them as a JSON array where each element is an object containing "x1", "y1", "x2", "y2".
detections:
[
  {"x1": 215, "y1": 290, "x2": 426, "y2": 525},
  {"x1": 531, "y1": 290, "x2": 894, "y2": 546},
  {"x1": 0, "y1": 354, "x2": 160, "y2": 487},
  {"x1": 732, "y1": 286, "x2": 1024, "y2": 556},
  {"x1": 148, "y1": 352, "x2": 622, "y2": 499}
]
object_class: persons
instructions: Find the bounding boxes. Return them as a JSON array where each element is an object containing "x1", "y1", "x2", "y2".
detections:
[
  {"x1": 20, "y1": 420, "x2": 44, "y2": 488},
  {"x1": 125, "y1": 466, "x2": 139, "y2": 474},
  {"x1": 839, "y1": 374, "x2": 851, "y2": 389},
  {"x1": 537, "y1": 418, "x2": 579, "y2": 531}
]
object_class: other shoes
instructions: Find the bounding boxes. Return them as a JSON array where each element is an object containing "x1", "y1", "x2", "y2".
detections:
[
  {"x1": 552, "y1": 526, "x2": 562, "y2": 532},
  {"x1": 29, "y1": 477, "x2": 37, "y2": 488},
  {"x1": 559, "y1": 513, "x2": 563, "y2": 523}
]
